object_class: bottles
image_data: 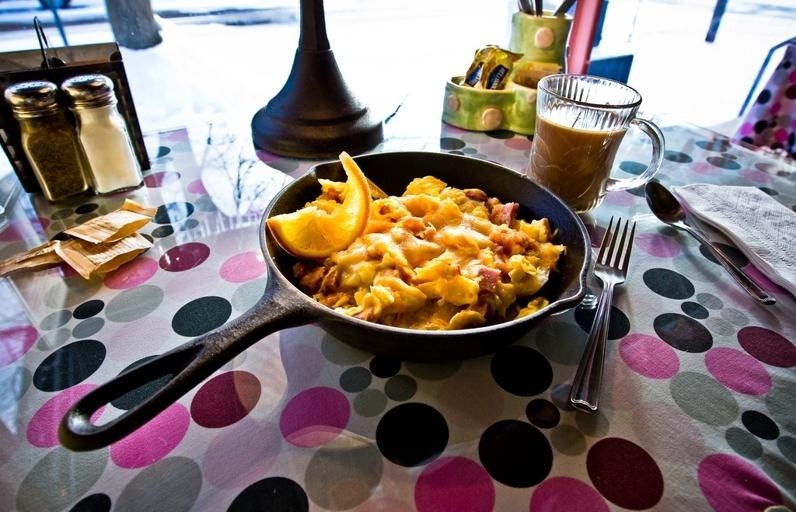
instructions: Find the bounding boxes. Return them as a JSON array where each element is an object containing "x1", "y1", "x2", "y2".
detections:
[
  {"x1": 3, "y1": 81, "x2": 89, "y2": 201},
  {"x1": 61, "y1": 73, "x2": 144, "y2": 196}
]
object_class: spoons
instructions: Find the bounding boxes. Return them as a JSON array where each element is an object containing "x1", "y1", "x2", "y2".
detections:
[{"x1": 644, "y1": 181, "x2": 777, "y2": 305}]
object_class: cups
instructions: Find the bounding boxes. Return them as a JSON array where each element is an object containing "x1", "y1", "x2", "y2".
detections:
[{"x1": 525, "y1": 74, "x2": 665, "y2": 214}]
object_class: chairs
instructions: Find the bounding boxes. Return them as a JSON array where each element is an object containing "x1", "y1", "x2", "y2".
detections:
[{"x1": 732, "y1": 37, "x2": 796, "y2": 161}]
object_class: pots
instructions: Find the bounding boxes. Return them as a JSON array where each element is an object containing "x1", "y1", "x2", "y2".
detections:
[{"x1": 58, "y1": 151, "x2": 591, "y2": 453}]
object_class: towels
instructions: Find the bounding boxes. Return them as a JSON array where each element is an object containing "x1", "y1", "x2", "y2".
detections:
[{"x1": 671, "y1": 183, "x2": 796, "y2": 297}]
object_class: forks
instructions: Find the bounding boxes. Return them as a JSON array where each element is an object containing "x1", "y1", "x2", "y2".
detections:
[{"x1": 569, "y1": 216, "x2": 638, "y2": 415}]
